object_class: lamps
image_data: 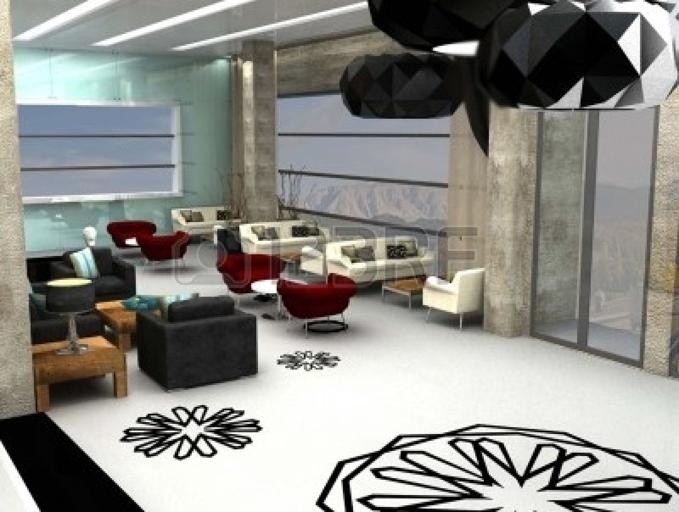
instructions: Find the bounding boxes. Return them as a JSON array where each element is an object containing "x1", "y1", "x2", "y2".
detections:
[{"x1": 328, "y1": 1, "x2": 679, "y2": 124}]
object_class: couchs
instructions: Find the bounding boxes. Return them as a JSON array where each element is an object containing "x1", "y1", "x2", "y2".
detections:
[{"x1": 24, "y1": 201, "x2": 488, "y2": 415}]
